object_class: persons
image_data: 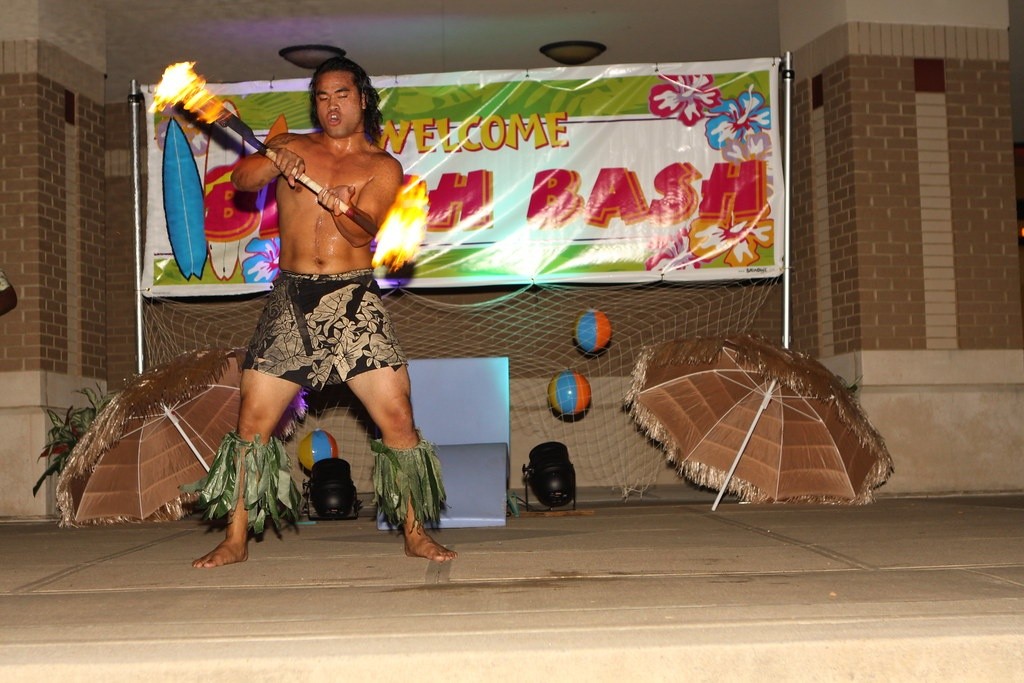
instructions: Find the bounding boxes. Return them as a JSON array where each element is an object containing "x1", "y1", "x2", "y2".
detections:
[{"x1": 192, "y1": 57, "x2": 458, "y2": 567}]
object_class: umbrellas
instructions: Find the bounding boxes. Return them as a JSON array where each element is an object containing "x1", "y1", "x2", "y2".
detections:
[
  {"x1": 622, "y1": 334, "x2": 893, "y2": 511},
  {"x1": 54, "y1": 342, "x2": 308, "y2": 528}
]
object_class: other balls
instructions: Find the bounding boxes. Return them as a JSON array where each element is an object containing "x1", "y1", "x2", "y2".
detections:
[
  {"x1": 297, "y1": 429, "x2": 338, "y2": 471},
  {"x1": 575, "y1": 309, "x2": 612, "y2": 353},
  {"x1": 549, "y1": 373, "x2": 593, "y2": 417}
]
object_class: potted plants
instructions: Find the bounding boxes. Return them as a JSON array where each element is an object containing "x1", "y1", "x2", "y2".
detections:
[{"x1": 32, "y1": 382, "x2": 114, "y2": 521}]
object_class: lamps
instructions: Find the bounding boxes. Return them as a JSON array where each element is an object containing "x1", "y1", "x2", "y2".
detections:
[
  {"x1": 279, "y1": 44, "x2": 346, "y2": 70},
  {"x1": 304, "y1": 457, "x2": 364, "y2": 522},
  {"x1": 521, "y1": 442, "x2": 577, "y2": 513},
  {"x1": 539, "y1": 39, "x2": 607, "y2": 67}
]
